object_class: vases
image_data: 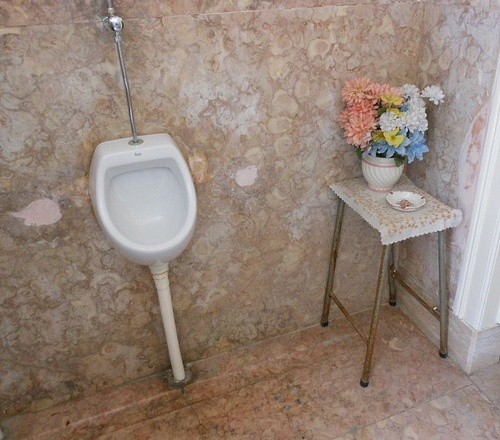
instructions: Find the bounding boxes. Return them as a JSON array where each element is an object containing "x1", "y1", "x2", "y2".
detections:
[{"x1": 359, "y1": 148, "x2": 408, "y2": 193}]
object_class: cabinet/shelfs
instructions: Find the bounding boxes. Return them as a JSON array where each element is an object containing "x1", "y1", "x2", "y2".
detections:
[{"x1": 319, "y1": 168, "x2": 462, "y2": 389}]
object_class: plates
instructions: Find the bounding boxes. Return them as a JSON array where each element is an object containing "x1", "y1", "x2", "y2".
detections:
[{"x1": 385, "y1": 189, "x2": 426, "y2": 213}]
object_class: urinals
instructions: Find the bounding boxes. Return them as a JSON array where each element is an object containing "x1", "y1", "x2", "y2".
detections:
[{"x1": 87, "y1": 132, "x2": 201, "y2": 269}]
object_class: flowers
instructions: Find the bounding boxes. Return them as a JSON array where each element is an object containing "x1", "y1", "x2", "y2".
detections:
[{"x1": 336, "y1": 76, "x2": 446, "y2": 165}]
